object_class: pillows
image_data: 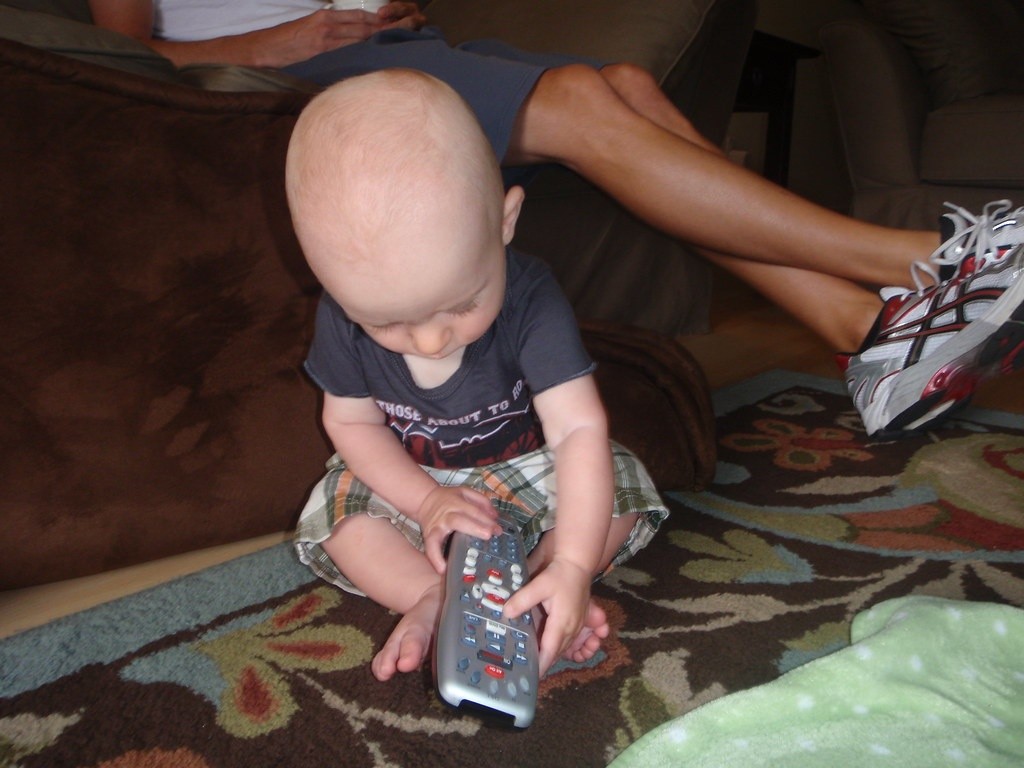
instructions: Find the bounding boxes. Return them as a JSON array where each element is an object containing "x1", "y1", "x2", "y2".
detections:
[{"x1": 875, "y1": 0, "x2": 1010, "y2": 101}]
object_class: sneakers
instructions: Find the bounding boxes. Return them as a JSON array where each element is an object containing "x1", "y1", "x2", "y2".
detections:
[
  {"x1": 833, "y1": 261, "x2": 1024, "y2": 442},
  {"x1": 931, "y1": 197, "x2": 1024, "y2": 283}
]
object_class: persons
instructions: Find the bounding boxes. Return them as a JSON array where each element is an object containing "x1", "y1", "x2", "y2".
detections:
[
  {"x1": 89, "y1": 1, "x2": 1024, "y2": 441},
  {"x1": 285, "y1": 69, "x2": 670, "y2": 681}
]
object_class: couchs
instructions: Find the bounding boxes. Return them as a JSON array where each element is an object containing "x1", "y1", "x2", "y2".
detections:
[
  {"x1": 824, "y1": 16, "x2": 1024, "y2": 232},
  {"x1": 0, "y1": 2, "x2": 758, "y2": 591}
]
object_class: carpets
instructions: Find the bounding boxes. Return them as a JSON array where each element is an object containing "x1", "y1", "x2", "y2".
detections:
[{"x1": 0, "y1": 366, "x2": 1024, "y2": 768}]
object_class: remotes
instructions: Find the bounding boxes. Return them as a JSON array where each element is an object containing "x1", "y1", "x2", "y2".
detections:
[{"x1": 431, "y1": 509, "x2": 540, "y2": 733}]
